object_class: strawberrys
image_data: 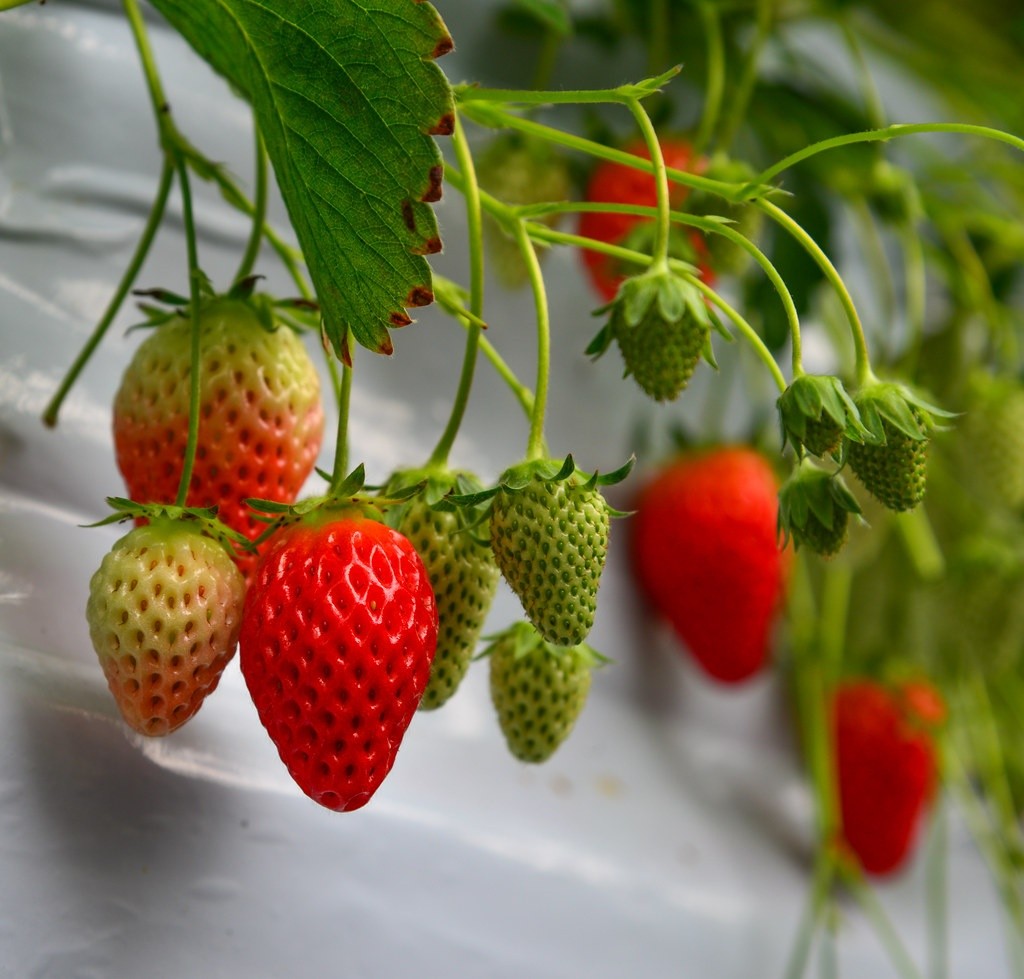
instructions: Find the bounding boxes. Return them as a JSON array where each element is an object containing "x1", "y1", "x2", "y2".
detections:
[{"x1": 80, "y1": 123, "x2": 1024, "y2": 876}]
